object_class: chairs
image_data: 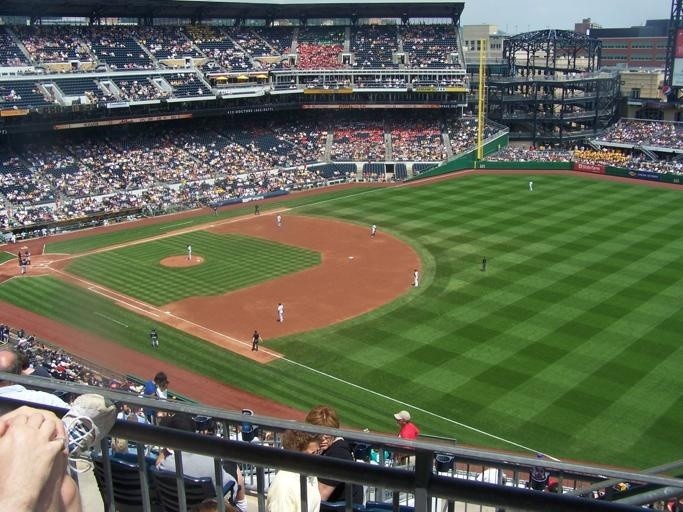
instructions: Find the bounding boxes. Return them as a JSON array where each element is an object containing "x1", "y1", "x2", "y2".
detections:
[
  {"x1": 2, "y1": 24, "x2": 505, "y2": 235},
  {"x1": 90, "y1": 452, "x2": 416, "y2": 512}
]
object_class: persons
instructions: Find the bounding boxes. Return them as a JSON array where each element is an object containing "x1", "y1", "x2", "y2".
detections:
[
  {"x1": 0, "y1": 23, "x2": 493, "y2": 244},
  {"x1": 493, "y1": 119, "x2": 681, "y2": 182},
  {"x1": 25, "y1": 251, "x2": 31, "y2": 264},
  {"x1": 528, "y1": 178, "x2": 533, "y2": 191},
  {"x1": 275, "y1": 302, "x2": 283, "y2": 322},
  {"x1": 411, "y1": 268, "x2": 419, "y2": 287},
  {"x1": 20, "y1": 258, "x2": 27, "y2": 274},
  {"x1": 0, "y1": 326, "x2": 683, "y2": 511},
  {"x1": 186, "y1": 245, "x2": 191, "y2": 260},
  {"x1": 482, "y1": 256, "x2": 486, "y2": 271}
]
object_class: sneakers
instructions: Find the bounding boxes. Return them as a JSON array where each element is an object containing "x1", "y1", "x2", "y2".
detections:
[{"x1": 60, "y1": 393, "x2": 117, "y2": 457}]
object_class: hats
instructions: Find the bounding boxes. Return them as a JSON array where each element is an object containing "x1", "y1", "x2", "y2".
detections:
[{"x1": 392, "y1": 410, "x2": 410, "y2": 421}]
